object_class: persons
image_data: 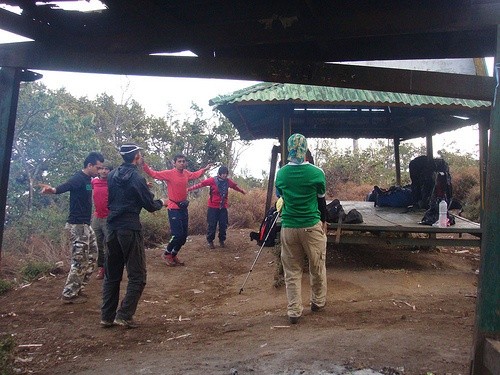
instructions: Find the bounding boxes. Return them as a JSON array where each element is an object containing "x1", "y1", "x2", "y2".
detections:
[
  {"x1": 90, "y1": 160, "x2": 113, "y2": 278},
  {"x1": 37, "y1": 152, "x2": 105, "y2": 303},
  {"x1": 188, "y1": 166, "x2": 246, "y2": 249},
  {"x1": 141, "y1": 156, "x2": 212, "y2": 266},
  {"x1": 100, "y1": 142, "x2": 170, "y2": 328},
  {"x1": 276, "y1": 132, "x2": 328, "y2": 322}
]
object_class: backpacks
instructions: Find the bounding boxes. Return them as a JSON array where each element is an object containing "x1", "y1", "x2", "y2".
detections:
[
  {"x1": 345, "y1": 209, "x2": 364, "y2": 224},
  {"x1": 325, "y1": 198, "x2": 346, "y2": 222},
  {"x1": 368, "y1": 187, "x2": 387, "y2": 202},
  {"x1": 250, "y1": 202, "x2": 282, "y2": 247},
  {"x1": 409, "y1": 156, "x2": 464, "y2": 216}
]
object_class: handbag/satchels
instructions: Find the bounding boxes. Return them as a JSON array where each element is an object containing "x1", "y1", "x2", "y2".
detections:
[
  {"x1": 419, "y1": 169, "x2": 456, "y2": 225},
  {"x1": 374, "y1": 183, "x2": 412, "y2": 207},
  {"x1": 178, "y1": 200, "x2": 189, "y2": 208}
]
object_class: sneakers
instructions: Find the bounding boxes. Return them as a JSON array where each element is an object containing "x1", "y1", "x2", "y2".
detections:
[
  {"x1": 171, "y1": 253, "x2": 184, "y2": 266},
  {"x1": 61, "y1": 291, "x2": 90, "y2": 304},
  {"x1": 161, "y1": 250, "x2": 176, "y2": 266}
]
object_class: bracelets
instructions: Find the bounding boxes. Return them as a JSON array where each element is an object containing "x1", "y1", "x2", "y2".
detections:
[
  {"x1": 160, "y1": 199, "x2": 164, "y2": 206},
  {"x1": 52, "y1": 188, "x2": 57, "y2": 194}
]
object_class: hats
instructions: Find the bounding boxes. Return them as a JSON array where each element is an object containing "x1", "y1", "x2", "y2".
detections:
[
  {"x1": 219, "y1": 167, "x2": 228, "y2": 176},
  {"x1": 119, "y1": 143, "x2": 143, "y2": 156},
  {"x1": 103, "y1": 161, "x2": 113, "y2": 171}
]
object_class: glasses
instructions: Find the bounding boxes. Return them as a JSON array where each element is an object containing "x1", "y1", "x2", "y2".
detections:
[{"x1": 178, "y1": 160, "x2": 186, "y2": 163}]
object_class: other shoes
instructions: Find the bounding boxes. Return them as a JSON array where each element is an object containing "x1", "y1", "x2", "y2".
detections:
[
  {"x1": 311, "y1": 301, "x2": 327, "y2": 312},
  {"x1": 95, "y1": 267, "x2": 105, "y2": 279},
  {"x1": 289, "y1": 312, "x2": 304, "y2": 323},
  {"x1": 219, "y1": 241, "x2": 225, "y2": 248},
  {"x1": 100, "y1": 320, "x2": 114, "y2": 328},
  {"x1": 208, "y1": 242, "x2": 215, "y2": 249},
  {"x1": 113, "y1": 315, "x2": 138, "y2": 328}
]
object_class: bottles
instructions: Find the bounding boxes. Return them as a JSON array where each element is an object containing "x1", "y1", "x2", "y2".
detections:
[{"x1": 439, "y1": 198, "x2": 447, "y2": 228}]
